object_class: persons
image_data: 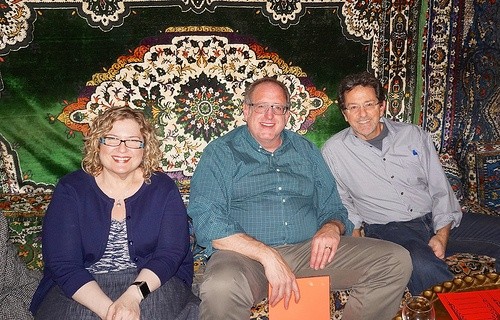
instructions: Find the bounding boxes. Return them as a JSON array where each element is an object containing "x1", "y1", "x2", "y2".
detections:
[
  {"x1": 186, "y1": 77, "x2": 416, "y2": 319},
  {"x1": 30, "y1": 106, "x2": 193, "y2": 320},
  {"x1": 321, "y1": 72, "x2": 499, "y2": 297}
]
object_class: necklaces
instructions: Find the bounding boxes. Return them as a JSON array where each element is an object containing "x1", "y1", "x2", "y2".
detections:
[{"x1": 97, "y1": 167, "x2": 144, "y2": 209}]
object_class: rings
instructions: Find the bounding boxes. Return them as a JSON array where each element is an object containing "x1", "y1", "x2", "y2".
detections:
[{"x1": 325, "y1": 246, "x2": 332, "y2": 250}]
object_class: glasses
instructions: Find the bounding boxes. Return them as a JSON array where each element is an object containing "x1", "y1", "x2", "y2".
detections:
[
  {"x1": 100, "y1": 137, "x2": 145, "y2": 149},
  {"x1": 249, "y1": 103, "x2": 289, "y2": 115},
  {"x1": 345, "y1": 102, "x2": 379, "y2": 112}
]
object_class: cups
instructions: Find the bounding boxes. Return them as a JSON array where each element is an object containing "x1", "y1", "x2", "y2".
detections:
[{"x1": 402, "y1": 295, "x2": 436, "y2": 320}]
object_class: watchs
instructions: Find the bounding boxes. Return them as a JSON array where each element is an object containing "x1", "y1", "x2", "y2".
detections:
[{"x1": 129, "y1": 280, "x2": 151, "y2": 302}]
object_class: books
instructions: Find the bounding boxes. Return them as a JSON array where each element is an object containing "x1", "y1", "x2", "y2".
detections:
[{"x1": 267, "y1": 274, "x2": 331, "y2": 320}]
